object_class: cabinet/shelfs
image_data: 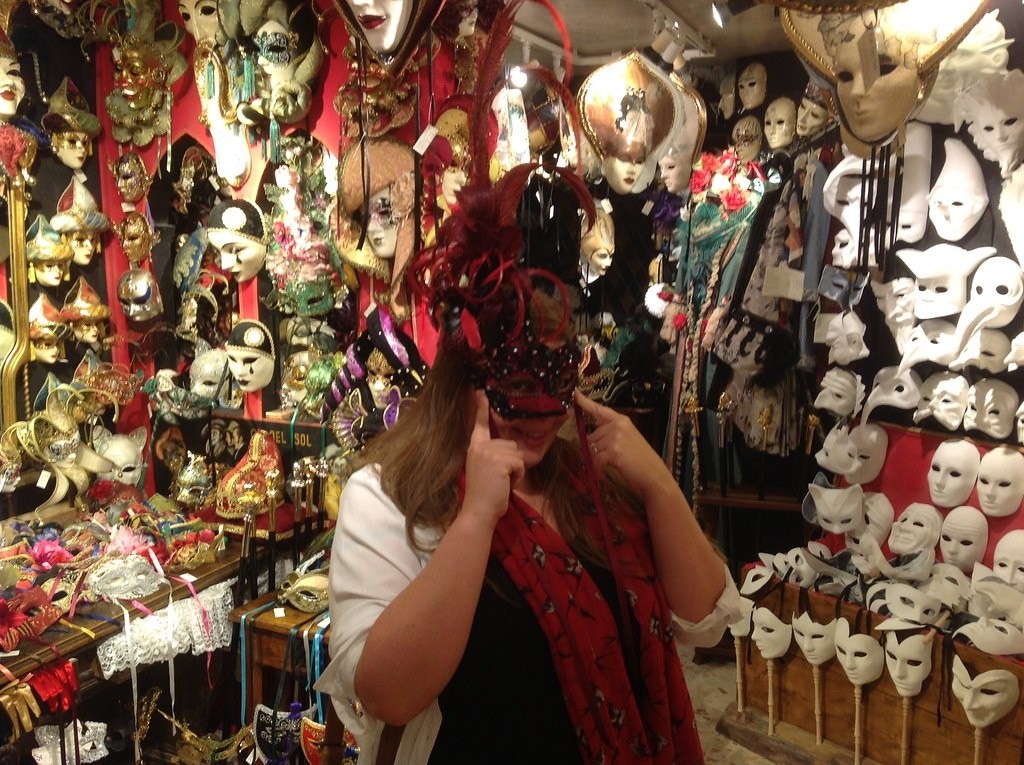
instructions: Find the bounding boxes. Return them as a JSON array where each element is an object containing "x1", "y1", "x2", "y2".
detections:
[{"x1": 687, "y1": 440, "x2": 827, "y2": 670}]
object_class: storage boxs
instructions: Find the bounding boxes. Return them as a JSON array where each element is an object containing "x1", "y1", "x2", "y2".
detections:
[{"x1": 715, "y1": 418, "x2": 1024, "y2": 765}]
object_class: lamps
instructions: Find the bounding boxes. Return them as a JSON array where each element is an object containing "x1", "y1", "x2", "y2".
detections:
[
  {"x1": 711, "y1": 0, "x2": 734, "y2": 31},
  {"x1": 644, "y1": 4, "x2": 696, "y2": 81}
]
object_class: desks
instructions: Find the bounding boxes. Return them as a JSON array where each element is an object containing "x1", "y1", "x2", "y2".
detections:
[
  {"x1": 224, "y1": 583, "x2": 345, "y2": 765},
  {"x1": 1, "y1": 504, "x2": 281, "y2": 689}
]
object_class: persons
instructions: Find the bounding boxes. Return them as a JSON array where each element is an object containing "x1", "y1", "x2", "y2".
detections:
[{"x1": 323, "y1": 247, "x2": 746, "y2": 764}]
